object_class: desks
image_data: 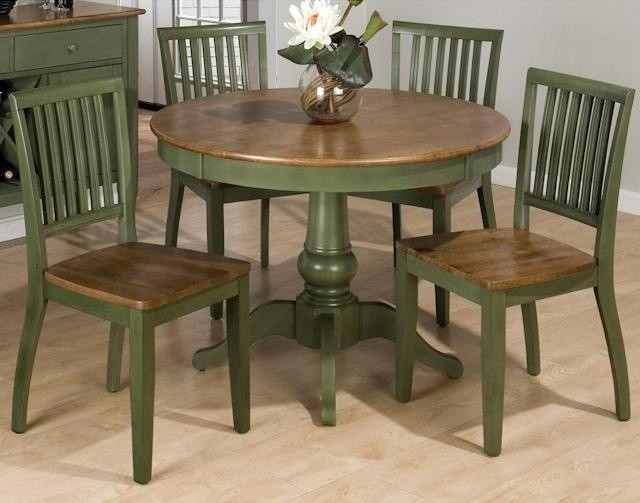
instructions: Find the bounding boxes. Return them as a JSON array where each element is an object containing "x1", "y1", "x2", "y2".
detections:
[{"x1": 149, "y1": 85, "x2": 512, "y2": 427}]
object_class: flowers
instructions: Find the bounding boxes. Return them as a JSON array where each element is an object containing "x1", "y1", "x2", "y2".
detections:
[{"x1": 275, "y1": 0, "x2": 388, "y2": 115}]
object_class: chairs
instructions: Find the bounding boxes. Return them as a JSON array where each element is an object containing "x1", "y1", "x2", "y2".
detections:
[
  {"x1": 156, "y1": 18, "x2": 312, "y2": 321},
  {"x1": 345, "y1": 19, "x2": 505, "y2": 328},
  {"x1": 391, "y1": 64, "x2": 636, "y2": 457},
  {"x1": 9, "y1": 74, "x2": 254, "y2": 483}
]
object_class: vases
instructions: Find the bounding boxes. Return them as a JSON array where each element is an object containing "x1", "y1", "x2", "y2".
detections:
[{"x1": 296, "y1": 62, "x2": 367, "y2": 125}]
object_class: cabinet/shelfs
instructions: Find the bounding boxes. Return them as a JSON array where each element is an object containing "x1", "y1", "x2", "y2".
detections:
[{"x1": 0, "y1": 0, "x2": 148, "y2": 210}]
